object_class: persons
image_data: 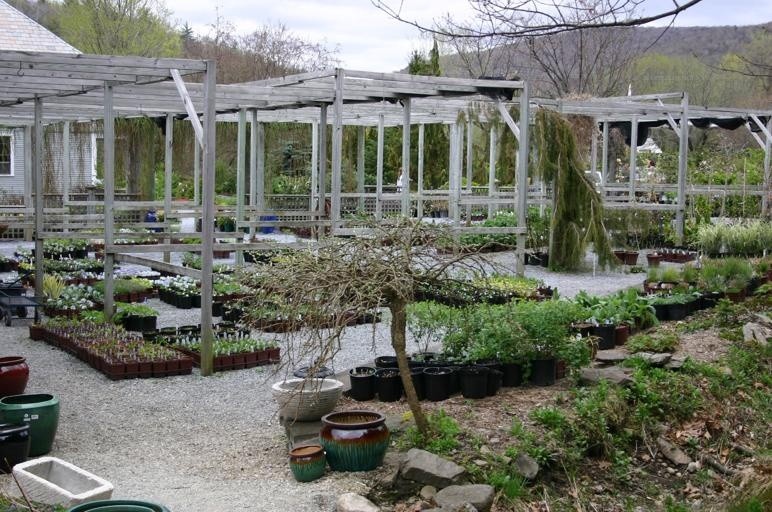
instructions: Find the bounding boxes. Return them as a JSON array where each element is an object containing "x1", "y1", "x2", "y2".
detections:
[
  {"x1": 620, "y1": 158, "x2": 657, "y2": 184},
  {"x1": 396, "y1": 167, "x2": 403, "y2": 193}
]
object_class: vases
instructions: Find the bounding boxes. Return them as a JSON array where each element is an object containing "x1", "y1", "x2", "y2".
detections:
[
  {"x1": 0, "y1": 392, "x2": 174, "y2": 512},
  {"x1": 290, "y1": 442, "x2": 328, "y2": 481},
  {"x1": 315, "y1": 404, "x2": 391, "y2": 470}
]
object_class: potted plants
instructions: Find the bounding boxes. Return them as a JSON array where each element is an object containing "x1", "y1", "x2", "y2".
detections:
[{"x1": 0, "y1": 185, "x2": 772, "y2": 427}]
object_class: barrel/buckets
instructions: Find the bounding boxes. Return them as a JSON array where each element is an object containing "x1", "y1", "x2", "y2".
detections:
[
  {"x1": 146, "y1": 209, "x2": 162, "y2": 233},
  {"x1": 176, "y1": 209, "x2": 195, "y2": 233},
  {"x1": 260, "y1": 216, "x2": 279, "y2": 234}
]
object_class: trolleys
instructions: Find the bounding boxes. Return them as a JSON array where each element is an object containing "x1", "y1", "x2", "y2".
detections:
[{"x1": 0, "y1": 269, "x2": 43, "y2": 327}]
